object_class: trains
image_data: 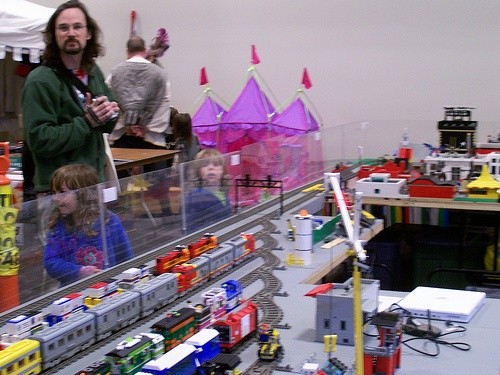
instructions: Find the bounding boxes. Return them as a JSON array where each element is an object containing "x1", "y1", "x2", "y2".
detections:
[{"x1": 0, "y1": 233, "x2": 257, "y2": 375}]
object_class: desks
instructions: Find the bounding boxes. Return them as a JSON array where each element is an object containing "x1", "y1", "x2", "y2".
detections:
[
  {"x1": 56, "y1": 195, "x2": 500, "y2": 375},
  {"x1": 111, "y1": 147, "x2": 181, "y2": 233}
]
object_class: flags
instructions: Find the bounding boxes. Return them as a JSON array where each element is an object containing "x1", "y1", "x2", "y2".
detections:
[
  {"x1": 252, "y1": 46, "x2": 259, "y2": 64},
  {"x1": 302, "y1": 70, "x2": 311, "y2": 89},
  {"x1": 200, "y1": 68, "x2": 208, "y2": 85}
]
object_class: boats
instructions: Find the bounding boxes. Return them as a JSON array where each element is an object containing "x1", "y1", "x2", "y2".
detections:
[{"x1": 356, "y1": 106, "x2": 500, "y2": 202}]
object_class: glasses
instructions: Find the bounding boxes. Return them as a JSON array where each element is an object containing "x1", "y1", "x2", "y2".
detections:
[{"x1": 55, "y1": 25, "x2": 90, "y2": 36}]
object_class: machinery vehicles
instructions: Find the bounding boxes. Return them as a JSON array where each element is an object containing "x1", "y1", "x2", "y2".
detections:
[{"x1": 257, "y1": 322, "x2": 282, "y2": 362}]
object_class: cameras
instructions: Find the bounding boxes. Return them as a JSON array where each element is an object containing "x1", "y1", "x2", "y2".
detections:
[{"x1": 91, "y1": 96, "x2": 118, "y2": 122}]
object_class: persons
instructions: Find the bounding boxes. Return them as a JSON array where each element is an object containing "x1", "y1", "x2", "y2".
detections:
[
  {"x1": 18, "y1": 0, "x2": 119, "y2": 304},
  {"x1": 166, "y1": 107, "x2": 192, "y2": 167},
  {"x1": 179, "y1": 148, "x2": 232, "y2": 229},
  {"x1": 105, "y1": 36, "x2": 176, "y2": 224},
  {"x1": 44, "y1": 163, "x2": 134, "y2": 288}
]
